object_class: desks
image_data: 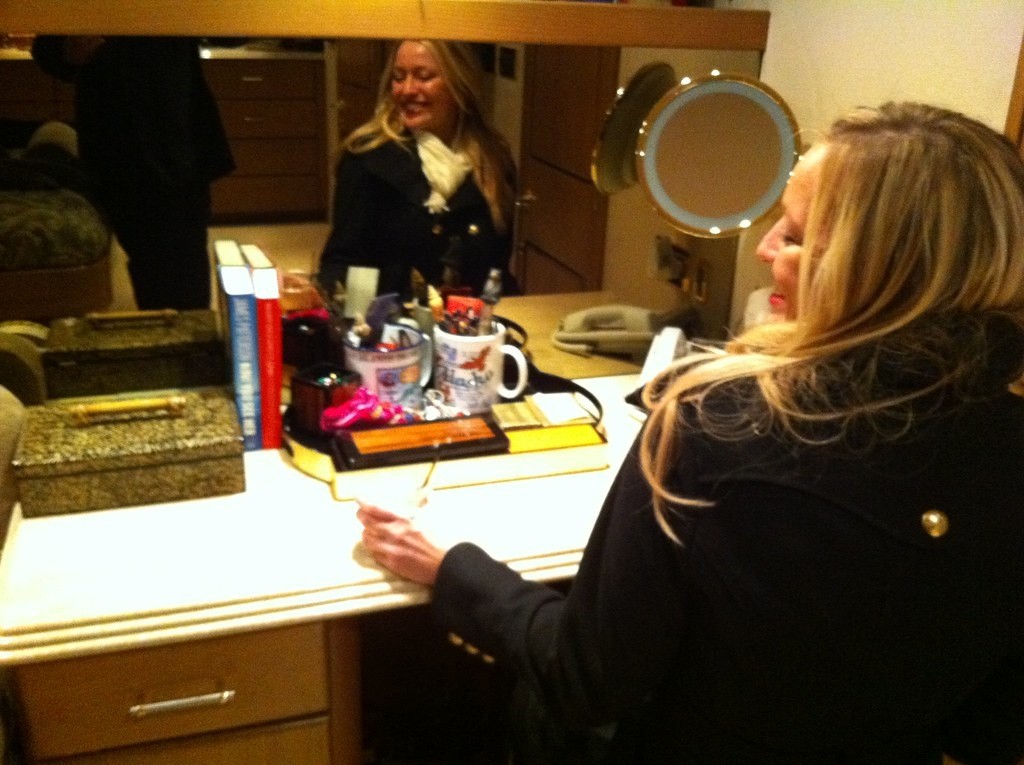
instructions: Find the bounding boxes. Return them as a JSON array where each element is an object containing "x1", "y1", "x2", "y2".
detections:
[{"x1": 0, "y1": 362, "x2": 673, "y2": 764}]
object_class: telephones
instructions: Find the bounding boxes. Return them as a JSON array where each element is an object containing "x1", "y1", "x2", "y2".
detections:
[
  {"x1": 552, "y1": 303, "x2": 696, "y2": 357},
  {"x1": 637, "y1": 326, "x2": 728, "y2": 393}
]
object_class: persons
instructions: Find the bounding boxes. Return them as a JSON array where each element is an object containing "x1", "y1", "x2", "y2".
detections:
[
  {"x1": 316, "y1": 39, "x2": 522, "y2": 306},
  {"x1": 358, "y1": 102, "x2": 1023, "y2": 765},
  {"x1": 29, "y1": 31, "x2": 232, "y2": 314}
]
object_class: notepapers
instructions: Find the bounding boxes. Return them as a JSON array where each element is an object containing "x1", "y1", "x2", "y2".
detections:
[
  {"x1": 490, "y1": 401, "x2": 540, "y2": 433},
  {"x1": 524, "y1": 392, "x2": 597, "y2": 429}
]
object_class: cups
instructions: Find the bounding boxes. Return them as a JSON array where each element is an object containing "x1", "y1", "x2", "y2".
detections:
[
  {"x1": 434, "y1": 316, "x2": 528, "y2": 404},
  {"x1": 342, "y1": 324, "x2": 432, "y2": 402},
  {"x1": 410, "y1": 293, "x2": 438, "y2": 338},
  {"x1": 278, "y1": 266, "x2": 311, "y2": 318}
]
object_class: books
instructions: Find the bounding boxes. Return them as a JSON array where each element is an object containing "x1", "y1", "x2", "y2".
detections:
[{"x1": 214, "y1": 238, "x2": 283, "y2": 452}]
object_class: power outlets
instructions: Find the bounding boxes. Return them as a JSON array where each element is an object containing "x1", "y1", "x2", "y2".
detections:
[{"x1": 653, "y1": 235, "x2": 712, "y2": 303}]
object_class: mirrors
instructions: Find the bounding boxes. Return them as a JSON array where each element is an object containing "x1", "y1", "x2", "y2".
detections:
[
  {"x1": 636, "y1": 71, "x2": 801, "y2": 242},
  {"x1": 1, "y1": 28, "x2": 764, "y2": 428}
]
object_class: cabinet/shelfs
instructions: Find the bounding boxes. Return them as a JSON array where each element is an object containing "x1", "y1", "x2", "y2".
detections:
[
  {"x1": 2, "y1": 594, "x2": 361, "y2": 765},
  {"x1": 197, "y1": 43, "x2": 335, "y2": 222}
]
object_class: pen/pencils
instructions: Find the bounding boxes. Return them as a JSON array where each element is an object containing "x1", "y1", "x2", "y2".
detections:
[{"x1": 435, "y1": 268, "x2": 502, "y2": 337}]
